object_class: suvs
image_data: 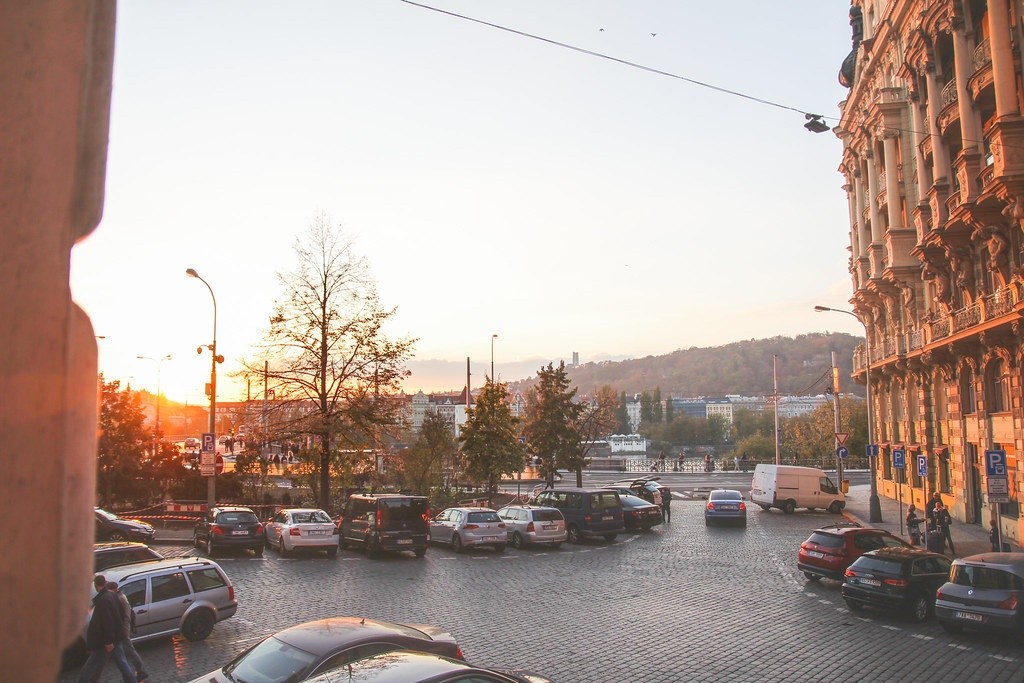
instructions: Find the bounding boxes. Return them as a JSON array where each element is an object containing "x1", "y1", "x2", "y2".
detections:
[
  {"x1": 797, "y1": 522, "x2": 916, "y2": 581},
  {"x1": 496, "y1": 505, "x2": 568, "y2": 549},
  {"x1": 63, "y1": 556, "x2": 238, "y2": 671}
]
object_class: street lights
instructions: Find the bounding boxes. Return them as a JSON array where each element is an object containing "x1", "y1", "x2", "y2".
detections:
[
  {"x1": 492, "y1": 335, "x2": 498, "y2": 420},
  {"x1": 186, "y1": 269, "x2": 224, "y2": 510},
  {"x1": 136, "y1": 354, "x2": 174, "y2": 493},
  {"x1": 815, "y1": 306, "x2": 882, "y2": 522}
]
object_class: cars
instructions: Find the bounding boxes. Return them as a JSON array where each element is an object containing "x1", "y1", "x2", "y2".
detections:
[
  {"x1": 701, "y1": 490, "x2": 748, "y2": 528},
  {"x1": 93, "y1": 542, "x2": 163, "y2": 573},
  {"x1": 934, "y1": 552, "x2": 1024, "y2": 633},
  {"x1": 219, "y1": 435, "x2": 231, "y2": 444},
  {"x1": 93, "y1": 506, "x2": 155, "y2": 543},
  {"x1": 841, "y1": 546, "x2": 953, "y2": 622},
  {"x1": 265, "y1": 508, "x2": 340, "y2": 555},
  {"x1": 194, "y1": 507, "x2": 267, "y2": 556},
  {"x1": 602, "y1": 475, "x2": 666, "y2": 532},
  {"x1": 184, "y1": 438, "x2": 201, "y2": 449},
  {"x1": 185, "y1": 617, "x2": 466, "y2": 683},
  {"x1": 430, "y1": 507, "x2": 507, "y2": 552},
  {"x1": 299, "y1": 649, "x2": 555, "y2": 683}
]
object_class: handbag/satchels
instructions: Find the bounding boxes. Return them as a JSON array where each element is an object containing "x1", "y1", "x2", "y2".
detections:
[{"x1": 130, "y1": 609, "x2": 136, "y2": 632}]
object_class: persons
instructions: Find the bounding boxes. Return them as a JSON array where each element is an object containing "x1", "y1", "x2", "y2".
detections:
[
  {"x1": 75, "y1": 575, "x2": 137, "y2": 683},
  {"x1": 660, "y1": 488, "x2": 672, "y2": 523},
  {"x1": 989, "y1": 520, "x2": 999, "y2": 552},
  {"x1": 268, "y1": 450, "x2": 294, "y2": 473},
  {"x1": 774, "y1": 451, "x2": 800, "y2": 466},
  {"x1": 649, "y1": 450, "x2": 729, "y2": 472},
  {"x1": 828, "y1": 448, "x2": 833, "y2": 463},
  {"x1": 926, "y1": 491, "x2": 956, "y2": 555},
  {"x1": 905, "y1": 504, "x2": 926, "y2": 545},
  {"x1": 91, "y1": 582, "x2": 149, "y2": 683},
  {"x1": 224, "y1": 436, "x2": 244, "y2": 453},
  {"x1": 184, "y1": 448, "x2": 223, "y2": 474},
  {"x1": 734, "y1": 451, "x2": 756, "y2": 472}
]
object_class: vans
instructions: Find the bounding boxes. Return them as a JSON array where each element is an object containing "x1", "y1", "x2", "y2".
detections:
[
  {"x1": 337, "y1": 493, "x2": 432, "y2": 557},
  {"x1": 533, "y1": 489, "x2": 627, "y2": 541}
]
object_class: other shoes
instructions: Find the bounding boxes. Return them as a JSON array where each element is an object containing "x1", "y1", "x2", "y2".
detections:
[{"x1": 134, "y1": 671, "x2": 149, "y2": 683}]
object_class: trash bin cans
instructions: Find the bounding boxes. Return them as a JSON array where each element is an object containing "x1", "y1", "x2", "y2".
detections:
[
  {"x1": 842, "y1": 480, "x2": 849, "y2": 493},
  {"x1": 927, "y1": 530, "x2": 945, "y2": 555}
]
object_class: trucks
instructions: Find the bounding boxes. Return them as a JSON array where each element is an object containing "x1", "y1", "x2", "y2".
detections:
[{"x1": 750, "y1": 463, "x2": 847, "y2": 513}]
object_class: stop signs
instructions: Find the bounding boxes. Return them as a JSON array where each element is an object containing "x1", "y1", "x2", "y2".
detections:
[{"x1": 215, "y1": 456, "x2": 223, "y2": 474}]
